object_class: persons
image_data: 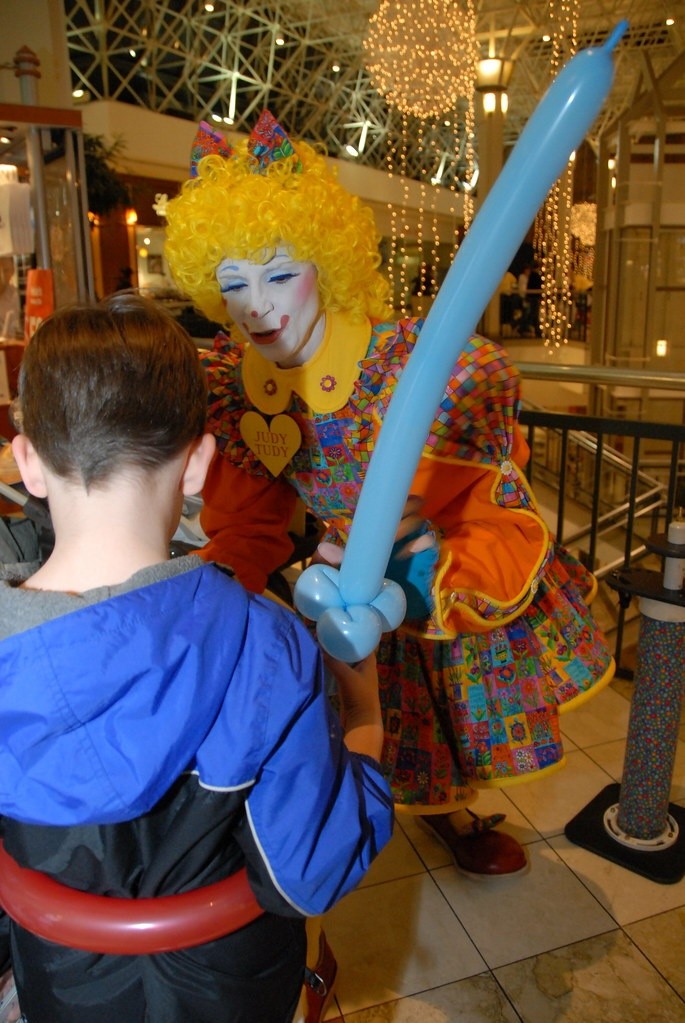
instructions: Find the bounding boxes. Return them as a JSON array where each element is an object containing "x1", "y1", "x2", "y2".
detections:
[
  {"x1": 498, "y1": 260, "x2": 546, "y2": 339},
  {"x1": 0, "y1": 257, "x2": 24, "y2": 338},
  {"x1": 0, "y1": 287, "x2": 395, "y2": 1023},
  {"x1": 153, "y1": 108, "x2": 616, "y2": 1023}
]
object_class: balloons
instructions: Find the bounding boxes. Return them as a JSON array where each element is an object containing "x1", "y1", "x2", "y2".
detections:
[
  {"x1": 0, "y1": 833, "x2": 263, "y2": 953},
  {"x1": 292, "y1": 19, "x2": 629, "y2": 696}
]
